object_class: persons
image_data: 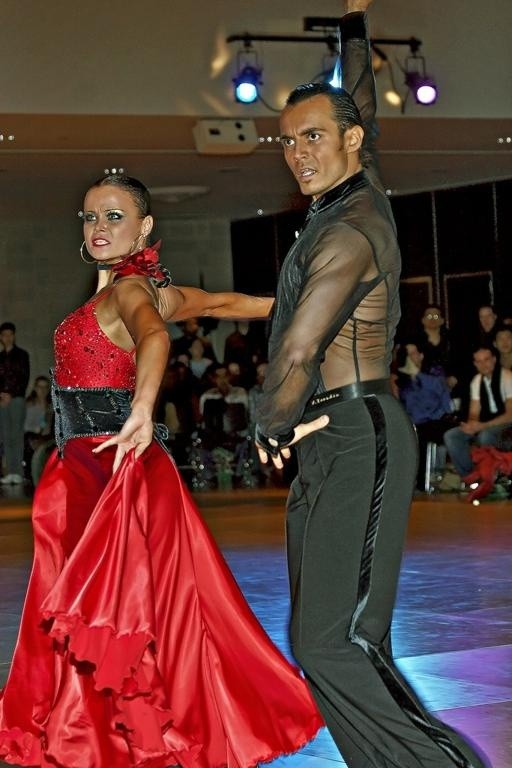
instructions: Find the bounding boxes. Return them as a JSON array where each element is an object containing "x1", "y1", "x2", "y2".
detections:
[
  {"x1": 390, "y1": 300, "x2": 512, "y2": 504},
  {"x1": 1, "y1": 169, "x2": 328, "y2": 767},
  {"x1": 0, "y1": 321, "x2": 31, "y2": 485},
  {"x1": 245, "y1": 0, "x2": 488, "y2": 767},
  {"x1": 150, "y1": 313, "x2": 300, "y2": 496},
  {"x1": 21, "y1": 375, "x2": 53, "y2": 490}
]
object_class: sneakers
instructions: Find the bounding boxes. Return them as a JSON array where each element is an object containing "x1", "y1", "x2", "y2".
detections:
[{"x1": 0, "y1": 474, "x2": 23, "y2": 484}]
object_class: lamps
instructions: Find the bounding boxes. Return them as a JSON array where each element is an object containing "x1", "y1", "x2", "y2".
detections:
[{"x1": 227, "y1": 16, "x2": 437, "y2": 115}]
